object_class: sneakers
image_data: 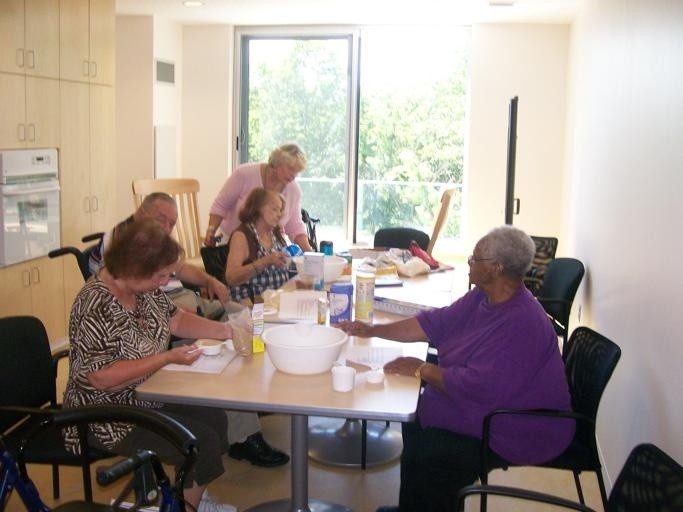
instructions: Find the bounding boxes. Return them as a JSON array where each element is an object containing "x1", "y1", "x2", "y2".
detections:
[{"x1": 197, "y1": 489, "x2": 237, "y2": 512}]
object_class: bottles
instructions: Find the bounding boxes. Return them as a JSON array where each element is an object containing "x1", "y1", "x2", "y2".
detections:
[
  {"x1": 303, "y1": 252, "x2": 325, "y2": 288},
  {"x1": 319, "y1": 299, "x2": 327, "y2": 323},
  {"x1": 355, "y1": 272, "x2": 376, "y2": 325},
  {"x1": 330, "y1": 283, "x2": 353, "y2": 325}
]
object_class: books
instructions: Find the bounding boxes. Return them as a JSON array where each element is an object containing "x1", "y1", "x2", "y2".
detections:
[{"x1": 373, "y1": 267, "x2": 402, "y2": 287}]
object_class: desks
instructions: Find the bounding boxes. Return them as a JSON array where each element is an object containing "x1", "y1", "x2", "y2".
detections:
[{"x1": 130, "y1": 250, "x2": 457, "y2": 512}]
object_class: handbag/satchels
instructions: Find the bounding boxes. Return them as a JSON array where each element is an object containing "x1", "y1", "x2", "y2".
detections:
[{"x1": 201, "y1": 245, "x2": 228, "y2": 273}]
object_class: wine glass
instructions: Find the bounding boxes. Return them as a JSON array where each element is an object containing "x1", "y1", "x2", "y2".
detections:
[{"x1": 367, "y1": 348, "x2": 385, "y2": 383}]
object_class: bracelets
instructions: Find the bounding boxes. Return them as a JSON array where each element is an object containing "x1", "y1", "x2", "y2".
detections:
[{"x1": 415, "y1": 363, "x2": 426, "y2": 378}]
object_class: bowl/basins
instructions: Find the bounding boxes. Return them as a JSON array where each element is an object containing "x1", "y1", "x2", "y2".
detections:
[
  {"x1": 261, "y1": 323, "x2": 348, "y2": 374},
  {"x1": 292, "y1": 255, "x2": 348, "y2": 283},
  {"x1": 194, "y1": 339, "x2": 222, "y2": 356}
]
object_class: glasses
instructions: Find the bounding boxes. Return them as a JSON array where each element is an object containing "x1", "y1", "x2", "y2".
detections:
[{"x1": 467, "y1": 254, "x2": 496, "y2": 266}]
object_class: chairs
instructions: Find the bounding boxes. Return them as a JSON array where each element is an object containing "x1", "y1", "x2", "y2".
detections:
[
  {"x1": 457, "y1": 441, "x2": 682, "y2": 511},
  {"x1": 533, "y1": 255, "x2": 587, "y2": 352},
  {"x1": 0, "y1": 313, "x2": 125, "y2": 511},
  {"x1": 131, "y1": 177, "x2": 209, "y2": 273},
  {"x1": 469, "y1": 236, "x2": 559, "y2": 292},
  {"x1": 478, "y1": 327, "x2": 621, "y2": 508},
  {"x1": 374, "y1": 228, "x2": 429, "y2": 250}
]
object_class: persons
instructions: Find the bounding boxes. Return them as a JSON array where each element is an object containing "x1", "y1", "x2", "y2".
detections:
[
  {"x1": 59, "y1": 218, "x2": 238, "y2": 512},
  {"x1": 224, "y1": 187, "x2": 292, "y2": 310},
  {"x1": 87, "y1": 192, "x2": 290, "y2": 467},
  {"x1": 336, "y1": 225, "x2": 576, "y2": 512},
  {"x1": 204, "y1": 143, "x2": 316, "y2": 251}
]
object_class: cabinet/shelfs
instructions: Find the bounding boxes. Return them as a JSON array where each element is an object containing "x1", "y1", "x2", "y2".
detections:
[
  {"x1": 57, "y1": 82, "x2": 119, "y2": 352},
  {"x1": 61, "y1": 0, "x2": 116, "y2": 87},
  {"x1": 0, "y1": 72, "x2": 57, "y2": 150},
  {"x1": 0, "y1": 252, "x2": 58, "y2": 354},
  {"x1": 0, "y1": 0, "x2": 60, "y2": 81}
]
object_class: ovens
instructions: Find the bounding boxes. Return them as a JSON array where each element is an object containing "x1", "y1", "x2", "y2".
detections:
[{"x1": 0, "y1": 148, "x2": 61, "y2": 269}]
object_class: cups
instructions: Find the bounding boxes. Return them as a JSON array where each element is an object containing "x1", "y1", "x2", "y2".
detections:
[
  {"x1": 320, "y1": 240, "x2": 334, "y2": 256},
  {"x1": 331, "y1": 366, "x2": 356, "y2": 392},
  {"x1": 282, "y1": 244, "x2": 303, "y2": 256}
]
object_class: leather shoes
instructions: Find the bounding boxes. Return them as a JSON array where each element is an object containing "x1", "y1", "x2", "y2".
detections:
[{"x1": 228, "y1": 432, "x2": 289, "y2": 467}]
